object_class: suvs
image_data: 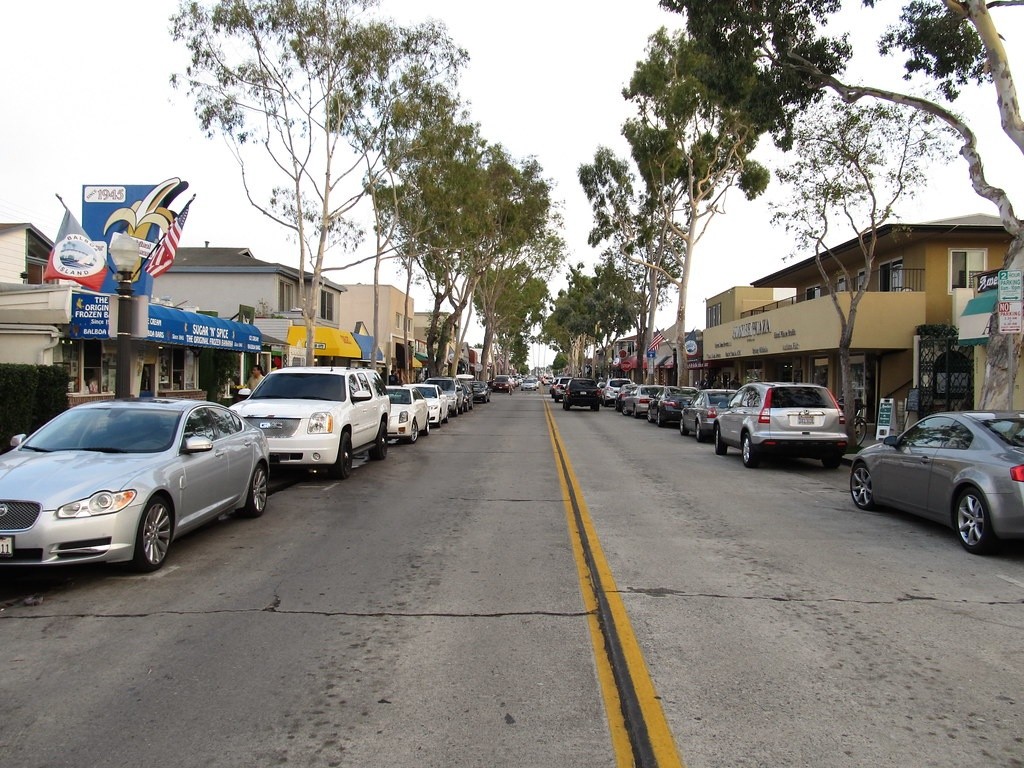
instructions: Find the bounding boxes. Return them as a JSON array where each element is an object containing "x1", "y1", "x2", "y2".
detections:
[
  {"x1": 600, "y1": 377, "x2": 634, "y2": 408},
  {"x1": 421, "y1": 376, "x2": 464, "y2": 415},
  {"x1": 560, "y1": 377, "x2": 601, "y2": 412},
  {"x1": 712, "y1": 381, "x2": 849, "y2": 471},
  {"x1": 622, "y1": 383, "x2": 666, "y2": 420}
]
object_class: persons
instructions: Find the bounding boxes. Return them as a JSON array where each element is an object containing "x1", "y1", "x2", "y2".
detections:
[
  {"x1": 507, "y1": 375, "x2": 515, "y2": 391},
  {"x1": 731, "y1": 376, "x2": 742, "y2": 390},
  {"x1": 711, "y1": 375, "x2": 724, "y2": 389},
  {"x1": 244, "y1": 365, "x2": 265, "y2": 395},
  {"x1": 700, "y1": 372, "x2": 713, "y2": 390},
  {"x1": 388, "y1": 370, "x2": 399, "y2": 385}
]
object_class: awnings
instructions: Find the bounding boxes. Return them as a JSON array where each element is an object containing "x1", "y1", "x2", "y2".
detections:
[
  {"x1": 415, "y1": 352, "x2": 437, "y2": 367},
  {"x1": 286, "y1": 324, "x2": 362, "y2": 359},
  {"x1": 957, "y1": 293, "x2": 1003, "y2": 350},
  {"x1": 350, "y1": 331, "x2": 384, "y2": 362},
  {"x1": 66, "y1": 293, "x2": 264, "y2": 354}
]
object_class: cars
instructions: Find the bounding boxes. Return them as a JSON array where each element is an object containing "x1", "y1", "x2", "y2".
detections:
[
  {"x1": 679, "y1": 389, "x2": 740, "y2": 445},
  {"x1": 380, "y1": 383, "x2": 430, "y2": 442},
  {"x1": 646, "y1": 386, "x2": 699, "y2": 429},
  {"x1": 553, "y1": 376, "x2": 574, "y2": 403},
  {"x1": 0, "y1": 396, "x2": 271, "y2": 574},
  {"x1": 615, "y1": 383, "x2": 640, "y2": 413},
  {"x1": 229, "y1": 364, "x2": 395, "y2": 480},
  {"x1": 459, "y1": 383, "x2": 473, "y2": 413},
  {"x1": 470, "y1": 380, "x2": 493, "y2": 403},
  {"x1": 403, "y1": 383, "x2": 449, "y2": 426},
  {"x1": 846, "y1": 412, "x2": 1024, "y2": 556},
  {"x1": 486, "y1": 373, "x2": 558, "y2": 394},
  {"x1": 593, "y1": 378, "x2": 606, "y2": 397}
]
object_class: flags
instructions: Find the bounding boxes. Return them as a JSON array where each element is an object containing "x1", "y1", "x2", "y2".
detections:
[
  {"x1": 447, "y1": 347, "x2": 456, "y2": 364},
  {"x1": 145, "y1": 203, "x2": 190, "y2": 279},
  {"x1": 43, "y1": 210, "x2": 109, "y2": 293},
  {"x1": 646, "y1": 332, "x2": 664, "y2": 353},
  {"x1": 680, "y1": 330, "x2": 702, "y2": 360},
  {"x1": 498, "y1": 357, "x2": 505, "y2": 365}
]
